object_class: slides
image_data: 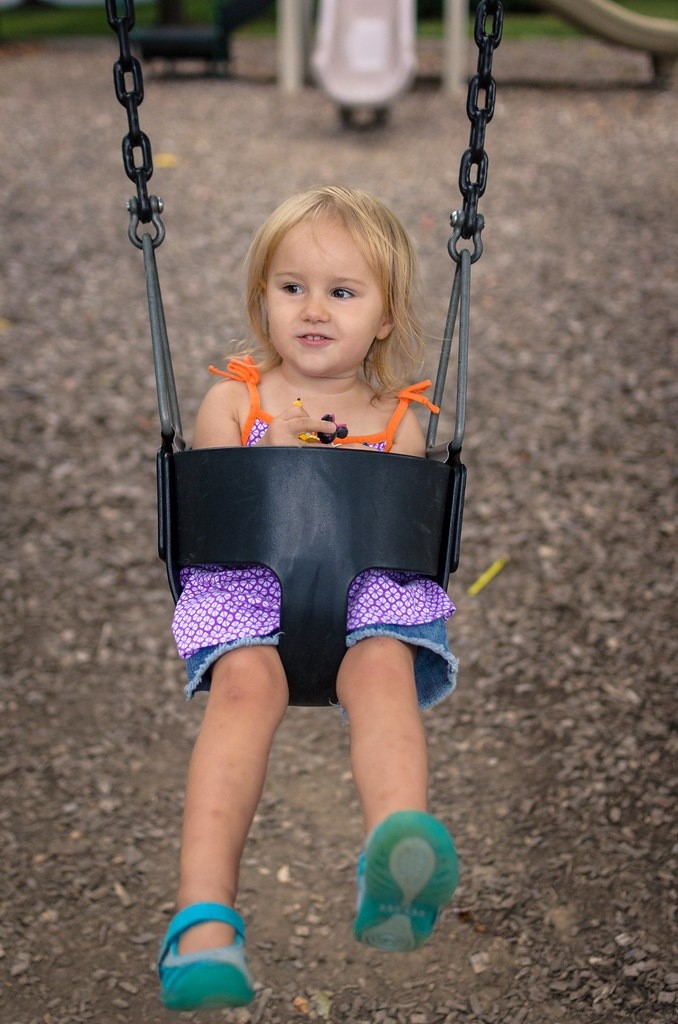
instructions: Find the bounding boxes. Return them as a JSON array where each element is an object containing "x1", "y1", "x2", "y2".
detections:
[
  {"x1": 311, "y1": 2, "x2": 418, "y2": 104},
  {"x1": 552, "y1": 0, "x2": 677, "y2": 52}
]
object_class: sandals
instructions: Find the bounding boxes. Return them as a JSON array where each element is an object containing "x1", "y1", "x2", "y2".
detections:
[
  {"x1": 352, "y1": 810, "x2": 458, "y2": 954},
  {"x1": 157, "y1": 902, "x2": 257, "y2": 1009}
]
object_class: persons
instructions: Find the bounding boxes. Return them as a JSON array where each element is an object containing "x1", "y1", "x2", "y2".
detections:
[{"x1": 155, "y1": 178, "x2": 459, "y2": 1008}]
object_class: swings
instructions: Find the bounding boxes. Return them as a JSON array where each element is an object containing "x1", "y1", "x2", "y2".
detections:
[{"x1": 104, "y1": 1, "x2": 502, "y2": 707}]
